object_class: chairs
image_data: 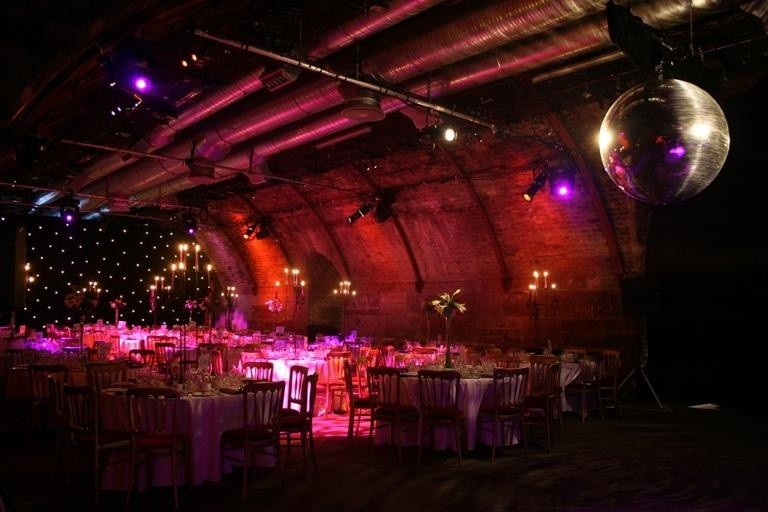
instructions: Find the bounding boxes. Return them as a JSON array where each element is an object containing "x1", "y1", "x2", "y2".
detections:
[
  {"x1": 318, "y1": 351, "x2": 351, "y2": 414},
  {"x1": 129, "y1": 350, "x2": 155, "y2": 368},
  {"x1": 479, "y1": 367, "x2": 529, "y2": 464},
  {"x1": 62, "y1": 385, "x2": 124, "y2": 509},
  {"x1": 602, "y1": 350, "x2": 619, "y2": 384},
  {"x1": 154, "y1": 343, "x2": 175, "y2": 365},
  {"x1": 523, "y1": 365, "x2": 559, "y2": 460},
  {"x1": 282, "y1": 366, "x2": 308, "y2": 457},
  {"x1": 220, "y1": 381, "x2": 285, "y2": 498},
  {"x1": 126, "y1": 386, "x2": 191, "y2": 509},
  {"x1": 368, "y1": 367, "x2": 418, "y2": 465},
  {"x1": 280, "y1": 366, "x2": 319, "y2": 481},
  {"x1": 374, "y1": 367, "x2": 523, "y2": 447},
  {"x1": 599, "y1": 352, "x2": 621, "y2": 420},
  {"x1": 242, "y1": 362, "x2": 274, "y2": 382},
  {"x1": 343, "y1": 360, "x2": 375, "y2": 437},
  {"x1": 416, "y1": 369, "x2": 464, "y2": 469}
]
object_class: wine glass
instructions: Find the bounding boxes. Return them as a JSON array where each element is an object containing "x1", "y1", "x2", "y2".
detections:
[
  {"x1": 152, "y1": 372, "x2": 159, "y2": 385},
  {"x1": 237, "y1": 376, "x2": 245, "y2": 391},
  {"x1": 136, "y1": 370, "x2": 144, "y2": 384}
]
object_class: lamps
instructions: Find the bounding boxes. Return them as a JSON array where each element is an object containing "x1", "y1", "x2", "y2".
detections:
[
  {"x1": 359, "y1": 194, "x2": 381, "y2": 216},
  {"x1": 348, "y1": 211, "x2": 362, "y2": 224},
  {"x1": 524, "y1": 159, "x2": 552, "y2": 201},
  {"x1": 244, "y1": 222, "x2": 258, "y2": 239}
]
